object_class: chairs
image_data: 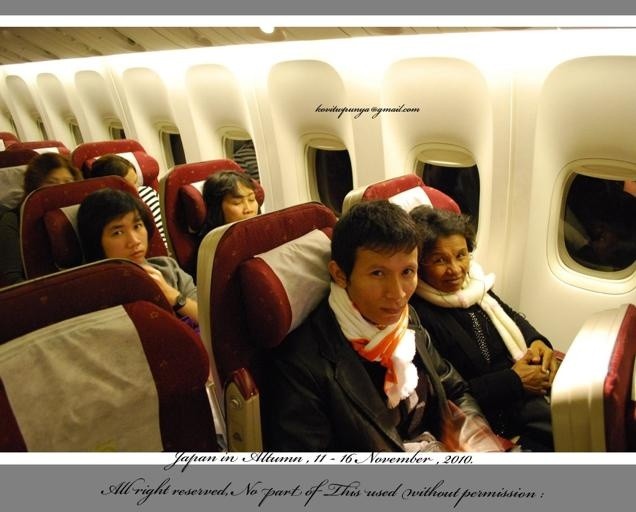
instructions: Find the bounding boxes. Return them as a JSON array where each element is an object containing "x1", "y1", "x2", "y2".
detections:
[{"x1": 0, "y1": 132, "x2": 636, "y2": 452}]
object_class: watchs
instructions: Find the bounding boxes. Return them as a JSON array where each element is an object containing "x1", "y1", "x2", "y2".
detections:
[{"x1": 171, "y1": 292, "x2": 187, "y2": 312}]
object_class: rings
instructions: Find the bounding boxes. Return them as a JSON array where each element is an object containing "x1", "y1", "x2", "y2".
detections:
[{"x1": 545, "y1": 370, "x2": 550, "y2": 377}]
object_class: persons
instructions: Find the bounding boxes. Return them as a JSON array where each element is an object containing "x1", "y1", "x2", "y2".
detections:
[
  {"x1": 1, "y1": 153, "x2": 84, "y2": 286},
  {"x1": 182, "y1": 169, "x2": 258, "y2": 284},
  {"x1": 408, "y1": 203, "x2": 560, "y2": 441},
  {"x1": 90, "y1": 154, "x2": 169, "y2": 250},
  {"x1": 79, "y1": 187, "x2": 199, "y2": 335},
  {"x1": 259, "y1": 200, "x2": 495, "y2": 452}
]
